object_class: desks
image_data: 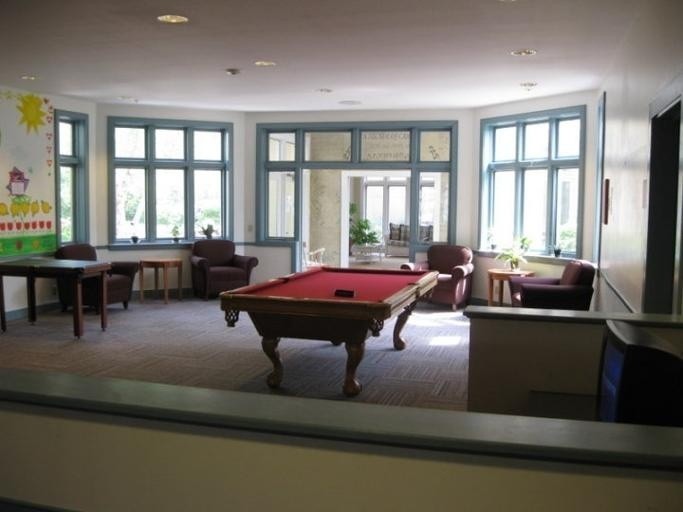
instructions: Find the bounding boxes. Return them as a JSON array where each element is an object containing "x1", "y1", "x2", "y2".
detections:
[
  {"x1": 487, "y1": 268, "x2": 535, "y2": 307},
  {"x1": 219, "y1": 265, "x2": 437, "y2": 399},
  {"x1": 352, "y1": 245, "x2": 386, "y2": 266},
  {"x1": 1, "y1": 257, "x2": 111, "y2": 337},
  {"x1": 138, "y1": 256, "x2": 183, "y2": 305},
  {"x1": 524, "y1": 388, "x2": 597, "y2": 424}
]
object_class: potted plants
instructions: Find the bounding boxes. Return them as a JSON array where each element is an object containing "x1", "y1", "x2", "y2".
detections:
[{"x1": 496, "y1": 235, "x2": 530, "y2": 271}]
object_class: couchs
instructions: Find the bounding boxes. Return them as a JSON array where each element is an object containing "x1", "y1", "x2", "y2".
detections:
[{"x1": 385, "y1": 223, "x2": 433, "y2": 257}]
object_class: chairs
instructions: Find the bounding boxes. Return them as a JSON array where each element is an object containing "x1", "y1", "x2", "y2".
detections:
[
  {"x1": 57, "y1": 243, "x2": 139, "y2": 314},
  {"x1": 300, "y1": 240, "x2": 327, "y2": 272},
  {"x1": 190, "y1": 238, "x2": 259, "y2": 301},
  {"x1": 508, "y1": 259, "x2": 597, "y2": 311},
  {"x1": 399, "y1": 242, "x2": 474, "y2": 314}
]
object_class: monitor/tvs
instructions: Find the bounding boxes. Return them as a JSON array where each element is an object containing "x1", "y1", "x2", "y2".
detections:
[{"x1": 595, "y1": 319, "x2": 683, "y2": 426}]
